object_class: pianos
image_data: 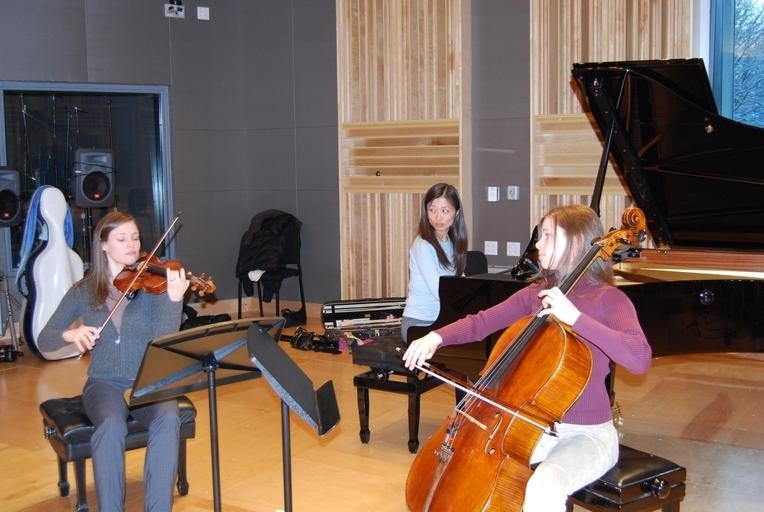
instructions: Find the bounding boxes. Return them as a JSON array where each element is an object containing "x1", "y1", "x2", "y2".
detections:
[{"x1": 406, "y1": 57, "x2": 764, "y2": 454}]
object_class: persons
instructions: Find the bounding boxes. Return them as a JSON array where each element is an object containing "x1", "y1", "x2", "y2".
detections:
[
  {"x1": 37, "y1": 211, "x2": 190, "y2": 512},
  {"x1": 401, "y1": 183, "x2": 467, "y2": 344}
]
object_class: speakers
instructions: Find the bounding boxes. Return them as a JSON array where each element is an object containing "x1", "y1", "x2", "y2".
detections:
[
  {"x1": 0, "y1": 166, "x2": 22, "y2": 228},
  {"x1": 71, "y1": 148, "x2": 116, "y2": 209}
]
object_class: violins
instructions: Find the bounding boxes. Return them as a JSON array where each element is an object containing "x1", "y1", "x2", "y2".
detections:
[{"x1": 114, "y1": 253, "x2": 216, "y2": 297}]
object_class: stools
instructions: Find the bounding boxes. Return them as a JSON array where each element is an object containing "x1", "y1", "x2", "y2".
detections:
[
  {"x1": 530, "y1": 441, "x2": 688, "y2": 511},
  {"x1": 353, "y1": 331, "x2": 470, "y2": 454},
  {"x1": 40, "y1": 390, "x2": 199, "y2": 511}
]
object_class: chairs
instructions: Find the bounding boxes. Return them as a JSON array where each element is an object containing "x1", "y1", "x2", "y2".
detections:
[{"x1": 235, "y1": 210, "x2": 306, "y2": 319}]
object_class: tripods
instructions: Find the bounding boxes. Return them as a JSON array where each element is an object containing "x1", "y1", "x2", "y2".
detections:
[{"x1": 1, "y1": 268, "x2": 23, "y2": 351}]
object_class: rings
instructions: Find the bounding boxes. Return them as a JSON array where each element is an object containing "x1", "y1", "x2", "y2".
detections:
[{"x1": 170, "y1": 279, "x2": 175, "y2": 281}]
